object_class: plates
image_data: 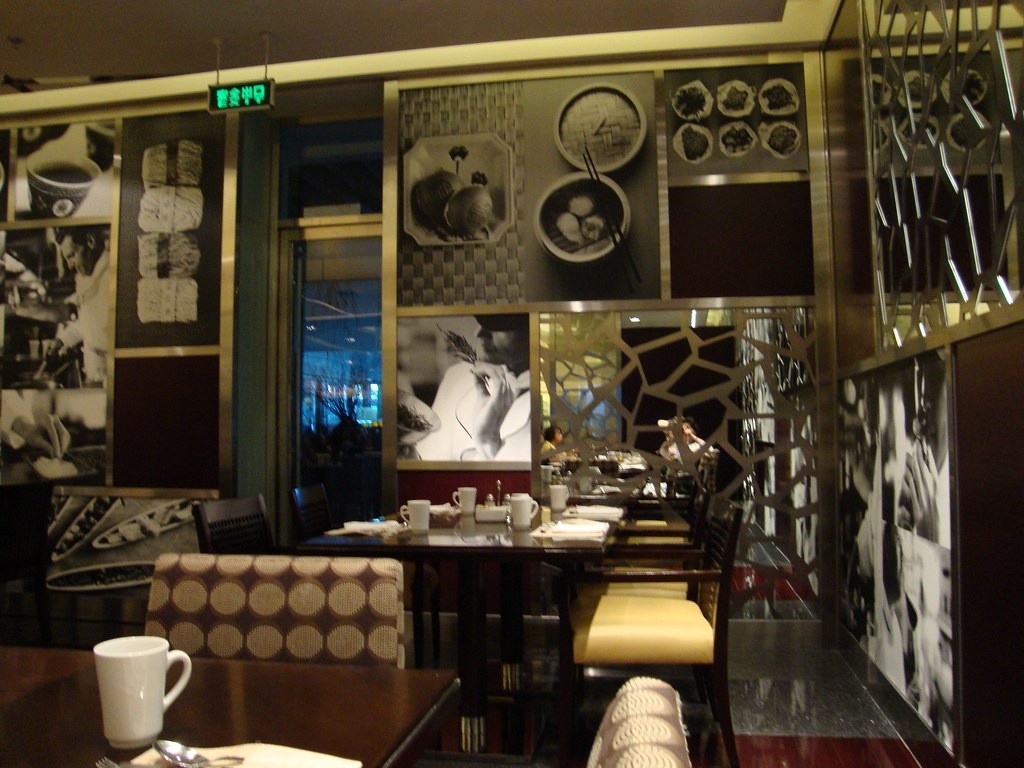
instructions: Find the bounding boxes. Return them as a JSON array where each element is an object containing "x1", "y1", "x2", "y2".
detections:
[
  {"x1": 21, "y1": 447, "x2": 97, "y2": 484},
  {"x1": 403, "y1": 133, "x2": 515, "y2": 245}
]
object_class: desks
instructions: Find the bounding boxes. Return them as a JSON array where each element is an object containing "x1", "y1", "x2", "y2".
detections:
[
  {"x1": 298, "y1": 505, "x2": 625, "y2": 704},
  {"x1": 0, "y1": 648, "x2": 460, "y2": 768}
]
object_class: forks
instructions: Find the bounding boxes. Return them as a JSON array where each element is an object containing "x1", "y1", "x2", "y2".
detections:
[{"x1": 95, "y1": 756, "x2": 119, "y2": 768}]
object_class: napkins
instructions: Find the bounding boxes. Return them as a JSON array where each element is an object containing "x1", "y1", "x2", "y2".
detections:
[
  {"x1": 343, "y1": 520, "x2": 399, "y2": 533},
  {"x1": 430, "y1": 503, "x2": 457, "y2": 516},
  {"x1": 562, "y1": 505, "x2": 624, "y2": 521},
  {"x1": 550, "y1": 519, "x2": 605, "y2": 533}
]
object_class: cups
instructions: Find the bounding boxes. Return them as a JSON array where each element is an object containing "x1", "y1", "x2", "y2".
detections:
[
  {"x1": 400, "y1": 499, "x2": 431, "y2": 535},
  {"x1": 541, "y1": 464, "x2": 553, "y2": 486},
  {"x1": 549, "y1": 484, "x2": 569, "y2": 511},
  {"x1": 27, "y1": 147, "x2": 101, "y2": 219},
  {"x1": 452, "y1": 487, "x2": 477, "y2": 515},
  {"x1": 93, "y1": 635, "x2": 192, "y2": 750},
  {"x1": 510, "y1": 493, "x2": 539, "y2": 531}
]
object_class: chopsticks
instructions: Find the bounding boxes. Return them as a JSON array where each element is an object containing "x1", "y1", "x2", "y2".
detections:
[{"x1": 582, "y1": 144, "x2": 644, "y2": 296}]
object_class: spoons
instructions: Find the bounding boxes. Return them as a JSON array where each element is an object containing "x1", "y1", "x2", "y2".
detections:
[{"x1": 153, "y1": 740, "x2": 244, "y2": 768}]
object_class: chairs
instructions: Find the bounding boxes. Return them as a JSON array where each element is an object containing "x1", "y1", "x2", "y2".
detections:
[
  {"x1": 565, "y1": 468, "x2": 740, "y2": 768},
  {"x1": 191, "y1": 493, "x2": 273, "y2": 555}
]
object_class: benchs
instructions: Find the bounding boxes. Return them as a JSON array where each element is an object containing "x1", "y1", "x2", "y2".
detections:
[{"x1": 144, "y1": 553, "x2": 407, "y2": 668}]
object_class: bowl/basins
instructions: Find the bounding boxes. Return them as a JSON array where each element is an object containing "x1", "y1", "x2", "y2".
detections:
[{"x1": 397, "y1": 387, "x2": 443, "y2": 446}]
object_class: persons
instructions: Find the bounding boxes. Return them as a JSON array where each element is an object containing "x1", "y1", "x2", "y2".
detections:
[
  {"x1": 658, "y1": 416, "x2": 714, "y2": 499},
  {"x1": 0, "y1": 389, "x2": 72, "y2": 463},
  {"x1": 847, "y1": 347, "x2": 954, "y2": 755},
  {"x1": 43, "y1": 224, "x2": 112, "y2": 391},
  {"x1": 540, "y1": 424, "x2": 581, "y2": 476},
  {"x1": 395, "y1": 312, "x2": 533, "y2": 463}
]
